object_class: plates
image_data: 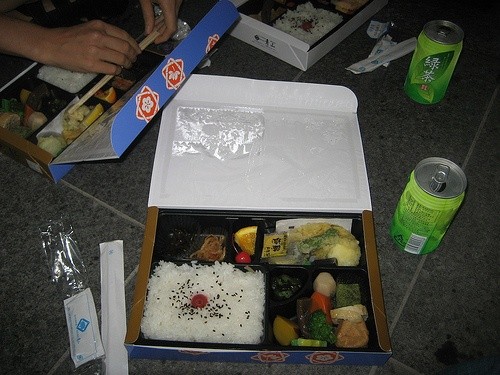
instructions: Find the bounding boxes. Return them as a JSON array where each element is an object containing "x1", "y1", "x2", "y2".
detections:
[
  {"x1": 134, "y1": 204, "x2": 387, "y2": 354},
  {"x1": 236, "y1": 0, "x2": 374, "y2": 52},
  {"x1": 0, "y1": 48, "x2": 169, "y2": 158}
]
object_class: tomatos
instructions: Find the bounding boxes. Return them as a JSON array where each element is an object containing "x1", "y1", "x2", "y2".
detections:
[{"x1": 235, "y1": 252, "x2": 252, "y2": 264}]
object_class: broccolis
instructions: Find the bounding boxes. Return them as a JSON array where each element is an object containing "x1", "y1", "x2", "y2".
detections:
[{"x1": 309, "y1": 310, "x2": 337, "y2": 345}]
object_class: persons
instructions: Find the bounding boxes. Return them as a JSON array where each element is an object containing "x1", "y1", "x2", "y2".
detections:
[{"x1": 0, "y1": 0, "x2": 184, "y2": 75}]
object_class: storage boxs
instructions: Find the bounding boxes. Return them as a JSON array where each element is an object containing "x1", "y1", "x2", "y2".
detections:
[
  {"x1": 0, "y1": 1, "x2": 242, "y2": 182},
  {"x1": 124, "y1": 73, "x2": 391, "y2": 366},
  {"x1": 224, "y1": 0, "x2": 392, "y2": 72}
]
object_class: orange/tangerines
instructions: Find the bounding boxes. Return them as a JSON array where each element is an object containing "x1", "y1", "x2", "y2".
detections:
[
  {"x1": 95, "y1": 87, "x2": 116, "y2": 103},
  {"x1": 235, "y1": 225, "x2": 258, "y2": 255}
]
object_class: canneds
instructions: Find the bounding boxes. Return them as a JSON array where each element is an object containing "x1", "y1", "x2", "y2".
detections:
[
  {"x1": 404, "y1": 19, "x2": 465, "y2": 104},
  {"x1": 391, "y1": 157, "x2": 467, "y2": 256}
]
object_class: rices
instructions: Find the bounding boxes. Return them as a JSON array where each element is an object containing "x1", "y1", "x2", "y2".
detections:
[
  {"x1": 37, "y1": 64, "x2": 97, "y2": 94},
  {"x1": 140, "y1": 261, "x2": 265, "y2": 345},
  {"x1": 273, "y1": 2, "x2": 343, "y2": 47}
]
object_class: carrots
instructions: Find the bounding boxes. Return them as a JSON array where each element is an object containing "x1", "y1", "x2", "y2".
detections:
[{"x1": 310, "y1": 291, "x2": 332, "y2": 324}]
